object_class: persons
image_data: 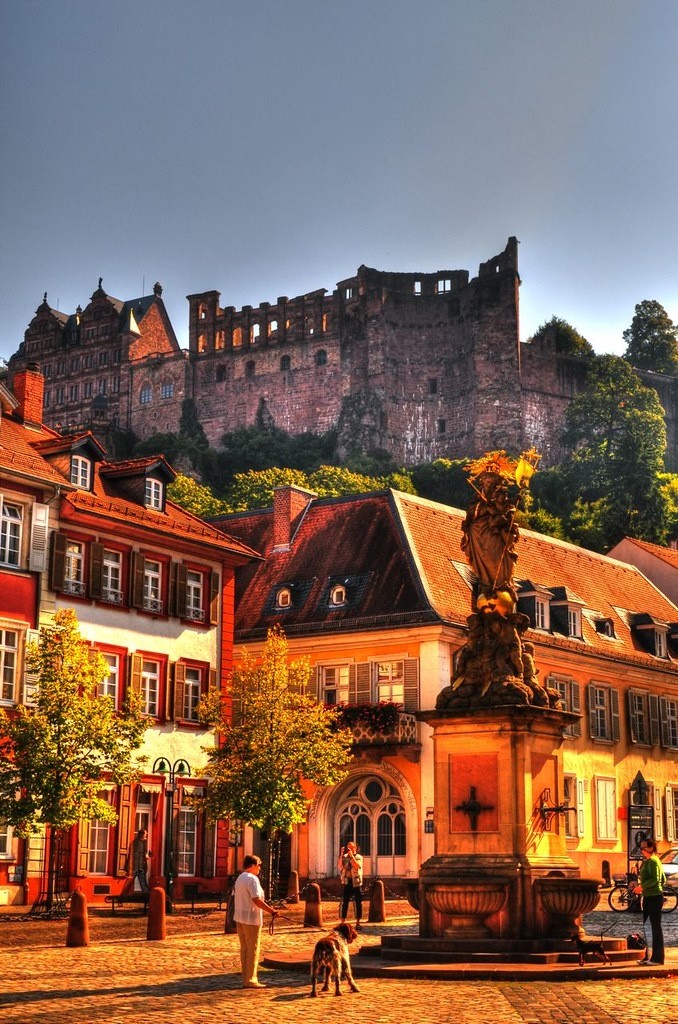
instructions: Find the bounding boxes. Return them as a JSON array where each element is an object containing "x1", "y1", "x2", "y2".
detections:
[
  {"x1": 458, "y1": 472, "x2": 521, "y2": 595},
  {"x1": 234, "y1": 855, "x2": 279, "y2": 987},
  {"x1": 632, "y1": 839, "x2": 666, "y2": 964},
  {"x1": 338, "y1": 842, "x2": 363, "y2": 930},
  {"x1": 134, "y1": 829, "x2": 148, "y2": 891}
]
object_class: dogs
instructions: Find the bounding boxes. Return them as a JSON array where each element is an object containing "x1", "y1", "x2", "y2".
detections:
[
  {"x1": 572, "y1": 931, "x2": 613, "y2": 966},
  {"x1": 310, "y1": 923, "x2": 360, "y2": 997}
]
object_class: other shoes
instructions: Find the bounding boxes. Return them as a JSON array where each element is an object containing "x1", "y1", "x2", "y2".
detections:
[
  {"x1": 356, "y1": 925, "x2": 363, "y2": 930},
  {"x1": 243, "y1": 981, "x2": 267, "y2": 988},
  {"x1": 637, "y1": 958, "x2": 664, "y2": 966}
]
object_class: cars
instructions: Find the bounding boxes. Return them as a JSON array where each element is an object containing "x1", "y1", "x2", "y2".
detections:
[{"x1": 655, "y1": 847, "x2": 678, "y2": 890}]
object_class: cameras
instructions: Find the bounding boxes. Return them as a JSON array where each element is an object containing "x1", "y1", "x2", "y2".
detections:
[{"x1": 344, "y1": 847, "x2": 349, "y2": 854}]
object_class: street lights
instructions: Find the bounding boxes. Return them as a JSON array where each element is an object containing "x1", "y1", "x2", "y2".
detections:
[{"x1": 153, "y1": 756, "x2": 192, "y2": 912}]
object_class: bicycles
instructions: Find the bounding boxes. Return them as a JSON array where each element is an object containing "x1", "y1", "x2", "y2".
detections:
[{"x1": 609, "y1": 873, "x2": 678, "y2": 913}]
